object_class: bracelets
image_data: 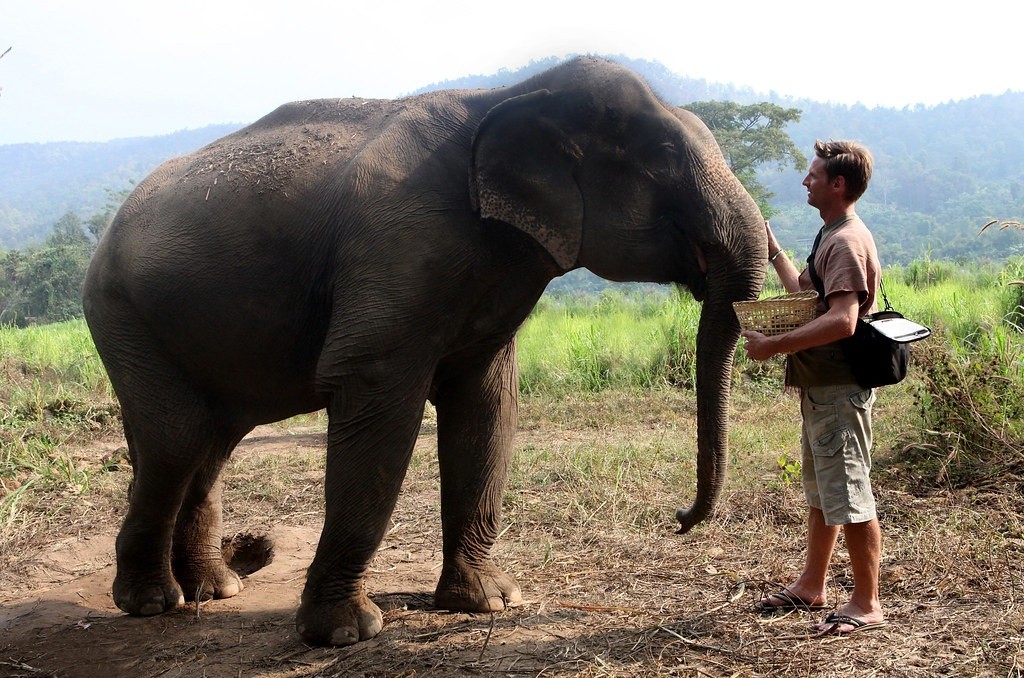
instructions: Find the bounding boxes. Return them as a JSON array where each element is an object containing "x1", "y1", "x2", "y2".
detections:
[{"x1": 768, "y1": 248, "x2": 783, "y2": 262}]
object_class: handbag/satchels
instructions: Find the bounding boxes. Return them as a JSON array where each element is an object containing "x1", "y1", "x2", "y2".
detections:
[{"x1": 847, "y1": 310, "x2": 932, "y2": 389}]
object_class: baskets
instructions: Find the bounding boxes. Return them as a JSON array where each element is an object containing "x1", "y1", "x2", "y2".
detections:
[{"x1": 732, "y1": 290, "x2": 819, "y2": 355}]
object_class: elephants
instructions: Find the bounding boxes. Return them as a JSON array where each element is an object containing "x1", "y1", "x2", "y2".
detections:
[{"x1": 80, "y1": 58, "x2": 768, "y2": 646}]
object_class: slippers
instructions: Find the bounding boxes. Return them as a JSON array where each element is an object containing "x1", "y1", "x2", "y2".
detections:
[
  {"x1": 819, "y1": 613, "x2": 886, "y2": 633},
  {"x1": 757, "y1": 592, "x2": 829, "y2": 610}
]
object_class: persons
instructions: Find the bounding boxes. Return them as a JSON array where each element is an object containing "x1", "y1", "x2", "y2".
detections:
[{"x1": 741, "y1": 141, "x2": 888, "y2": 636}]
